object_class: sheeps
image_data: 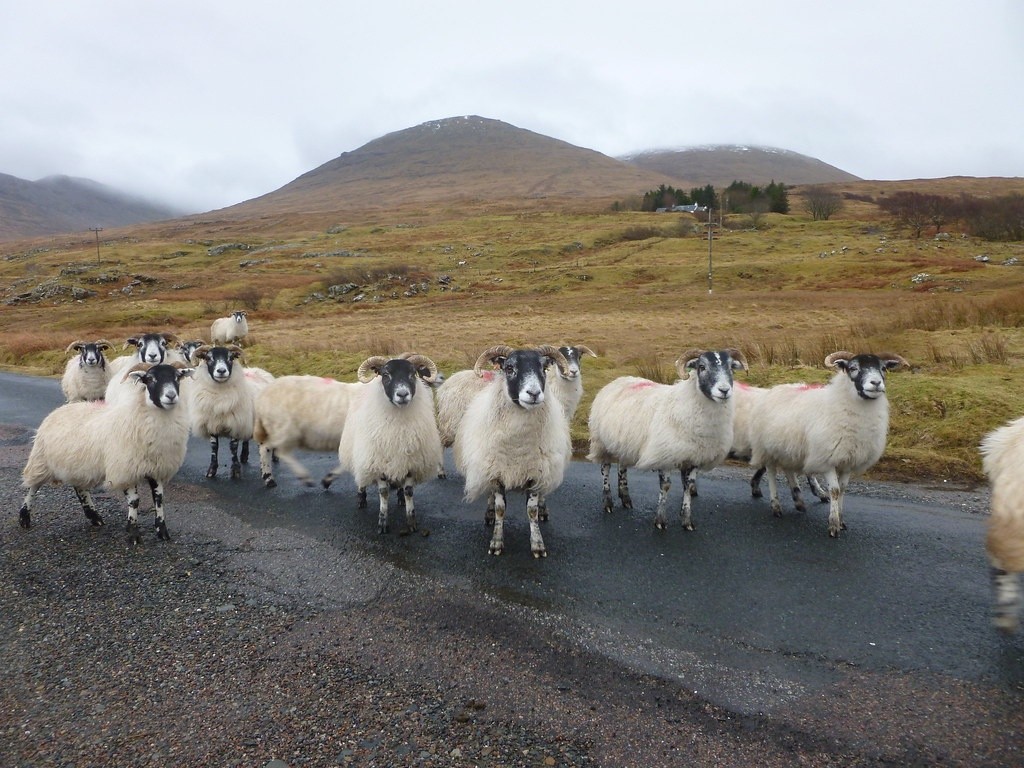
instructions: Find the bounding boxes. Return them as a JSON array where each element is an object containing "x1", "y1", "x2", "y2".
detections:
[
  {"x1": 253, "y1": 349, "x2": 439, "y2": 534},
  {"x1": 17, "y1": 361, "x2": 189, "y2": 545},
  {"x1": 974, "y1": 413, "x2": 1024, "y2": 630},
  {"x1": 434, "y1": 342, "x2": 597, "y2": 559},
  {"x1": 210, "y1": 309, "x2": 248, "y2": 349},
  {"x1": 61, "y1": 331, "x2": 275, "y2": 480},
  {"x1": 588, "y1": 345, "x2": 911, "y2": 536}
]
